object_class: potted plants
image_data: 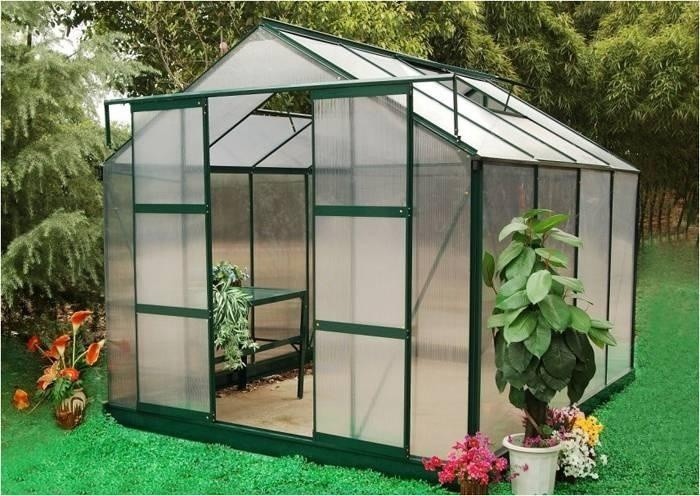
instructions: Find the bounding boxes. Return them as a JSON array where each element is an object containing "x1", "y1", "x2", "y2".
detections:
[
  {"x1": 208, "y1": 258, "x2": 263, "y2": 374},
  {"x1": 481, "y1": 202, "x2": 621, "y2": 496}
]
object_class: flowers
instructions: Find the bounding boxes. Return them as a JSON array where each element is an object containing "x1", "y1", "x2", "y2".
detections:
[
  {"x1": 10, "y1": 309, "x2": 108, "y2": 423},
  {"x1": 523, "y1": 404, "x2": 610, "y2": 487},
  {"x1": 417, "y1": 427, "x2": 531, "y2": 491},
  {"x1": 505, "y1": 423, "x2": 561, "y2": 448}
]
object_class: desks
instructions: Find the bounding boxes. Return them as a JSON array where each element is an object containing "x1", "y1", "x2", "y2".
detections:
[{"x1": 212, "y1": 284, "x2": 311, "y2": 401}]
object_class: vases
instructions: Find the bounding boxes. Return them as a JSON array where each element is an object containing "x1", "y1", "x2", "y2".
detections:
[
  {"x1": 46, "y1": 386, "x2": 88, "y2": 432},
  {"x1": 455, "y1": 470, "x2": 490, "y2": 495}
]
